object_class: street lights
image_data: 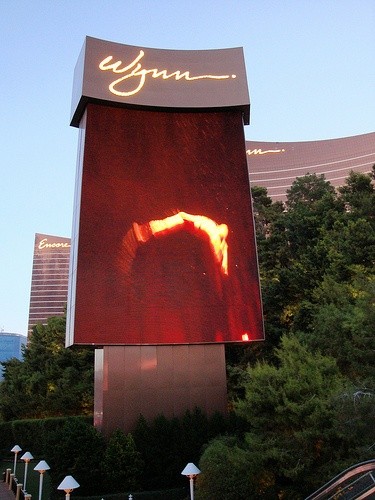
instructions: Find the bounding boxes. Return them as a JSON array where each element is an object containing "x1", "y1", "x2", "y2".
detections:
[
  {"x1": 11, "y1": 445, "x2": 21, "y2": 473},
  {"x1": 57, "y1": 476, "x2": 80, "y2": 499},
  {"x1": 20, "y1": 452, "x2": 34, "y2": 489},
  {"x1": 35, "y1": 460, "x2": 50, "y2": 499},
  {"x1": 182, "y1": 463, "x2": 201, "y2": 499}
]
